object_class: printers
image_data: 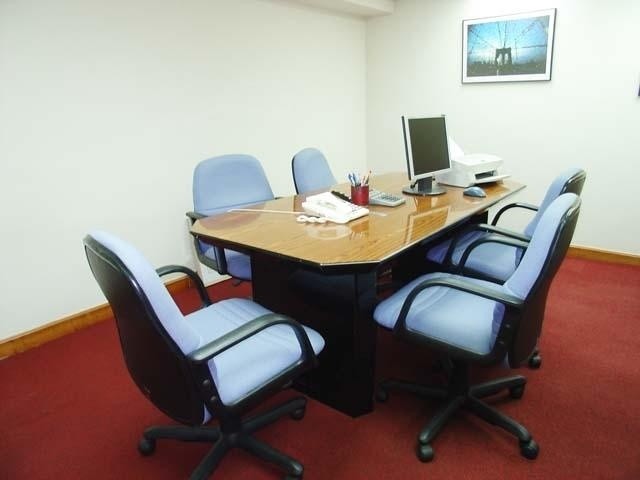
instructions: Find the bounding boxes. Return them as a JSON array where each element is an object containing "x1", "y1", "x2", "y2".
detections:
[{"x1": 435, "y1": 152, "x2": 511, "y2": 188}]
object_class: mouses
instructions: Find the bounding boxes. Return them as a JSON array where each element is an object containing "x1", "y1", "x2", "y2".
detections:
[{"x1": 463, "y1": 186, "x2": 487, "y2": 197}]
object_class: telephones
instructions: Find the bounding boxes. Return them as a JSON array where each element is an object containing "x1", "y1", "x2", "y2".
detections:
[{"x1": 302, "y1": 192, "x2": 369, "y2": 224}]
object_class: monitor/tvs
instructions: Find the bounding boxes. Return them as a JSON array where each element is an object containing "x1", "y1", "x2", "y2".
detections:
[{"x1": 402, "y1": 112, "x2": 453, "y2": 196}]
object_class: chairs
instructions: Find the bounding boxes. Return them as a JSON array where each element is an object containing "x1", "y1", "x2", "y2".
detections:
[
  {"x1": 186, "y1": 153, "x2": 274, "y2": 284},
  {"x1": 84, "y1": 232, "x2": 326, "y2": 480},
  {"x1": 426, "y1": 167, "x2": 586, "y2": 370},
  {"x1": 373, "y1": 192, "x2": 582, "y2": 463},
  {"x1": 292, "y1": 146, "x2": 336, "y2": 197}
]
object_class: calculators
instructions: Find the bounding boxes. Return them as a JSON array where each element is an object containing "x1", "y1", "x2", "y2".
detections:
[{"x1": 368, "y1": 188, "x2": 405, "y2": 206}]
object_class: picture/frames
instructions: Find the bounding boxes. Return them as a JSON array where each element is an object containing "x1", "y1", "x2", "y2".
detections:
[{"x1": 460, "y1": 6, "x2": 558, "y2": 86}]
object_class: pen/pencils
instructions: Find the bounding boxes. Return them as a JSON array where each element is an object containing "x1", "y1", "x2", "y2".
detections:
[{"x1": 349, "y1": 170, "x2": 372, "y2": 186}]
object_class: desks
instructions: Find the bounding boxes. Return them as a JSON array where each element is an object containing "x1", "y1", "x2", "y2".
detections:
[{"x1": 187, "y1": 172, "x2": 525, "y2": 419}]
